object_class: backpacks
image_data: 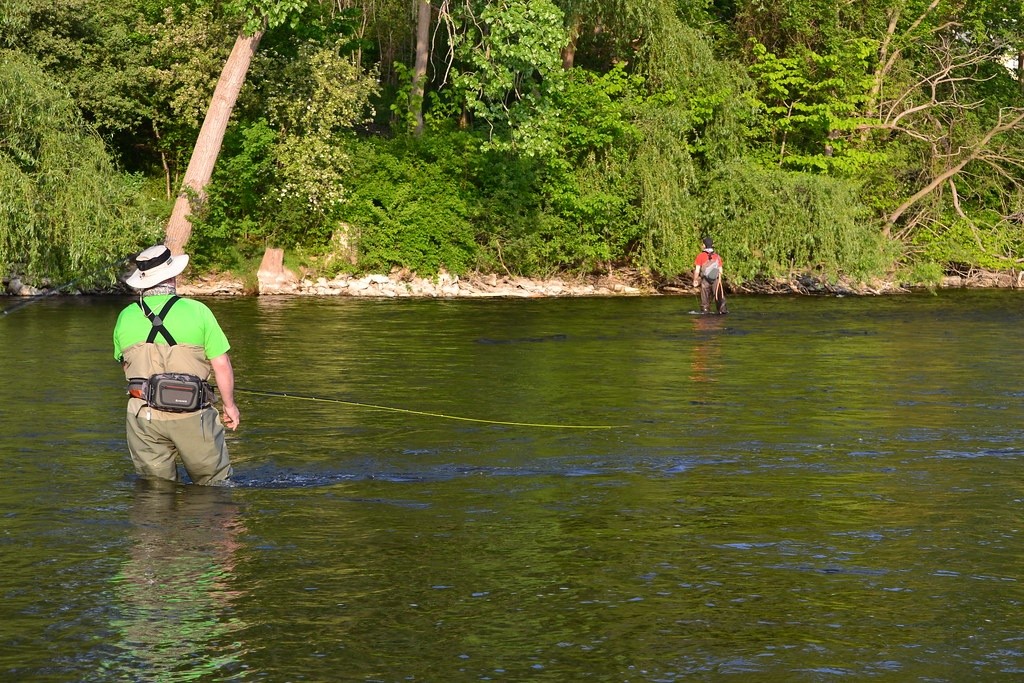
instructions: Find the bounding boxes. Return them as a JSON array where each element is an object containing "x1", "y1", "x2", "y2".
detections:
[{"x1": 702, "y1": 250, "x2": 721, "y2": 282}]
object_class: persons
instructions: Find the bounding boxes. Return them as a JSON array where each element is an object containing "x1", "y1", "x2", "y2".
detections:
[
  {"x1": 112, "y1": 245, "x2": 240, "y2": 484},
  {"x1": 693, "y1": 237, "x2": 727, "y2": 313}
]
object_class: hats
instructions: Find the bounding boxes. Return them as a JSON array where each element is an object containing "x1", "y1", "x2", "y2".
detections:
[{"x1": 126, "y1": 244, "x2": 189, "y2": 288}]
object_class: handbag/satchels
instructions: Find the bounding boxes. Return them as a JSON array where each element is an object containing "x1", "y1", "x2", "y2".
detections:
[{"x1": 129, "y1": 372, "x2": 216, "y2": 412}]
